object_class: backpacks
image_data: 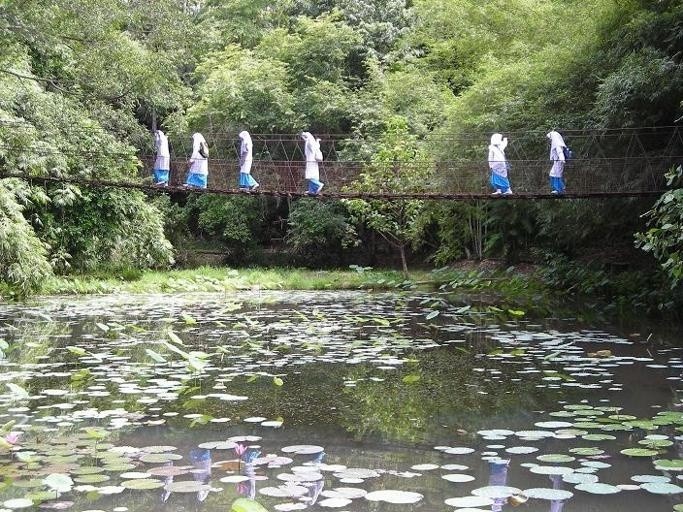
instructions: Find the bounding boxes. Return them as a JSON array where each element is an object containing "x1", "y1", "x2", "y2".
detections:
[{"x1": 199, "y1": 142, "x2": 210, "y2": 158}]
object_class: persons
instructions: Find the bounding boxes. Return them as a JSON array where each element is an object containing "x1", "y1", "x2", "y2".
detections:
[
  {"x1": 301, "y1": 131, "x2": 324, "y2": 194},
  {"x1": 488, "y1": 133, "x2": 512, "y2": 194},
  {"x1": 183, "y1": 132, "x2": 209, "y2": 188},
  {"x1": 162, "y1": 449, "x2": 325, "y2": 506},
  {"x1": 239, "y1": 131, "x2": 259, "y2": 190},
  {"x1": 550, "y1": 474, "x2": 565, "y2": 511},
  {"x1": 153, "y1": 130, "x2": 170, "y2": 187},
  {"x1": 546, "y1": 131, "x2": 567, "y2": 194},
  {"x1": 488, "y1": 458, "x2": 508, "y2": 512}
]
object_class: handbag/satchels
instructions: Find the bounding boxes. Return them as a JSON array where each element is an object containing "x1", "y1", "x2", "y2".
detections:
[
  {"x1": 506, "y1": 161, "x2": 513, "y2": 174},
  {"x1": 563, "y1": 146, "x2": 573, "y2": 162}
]
